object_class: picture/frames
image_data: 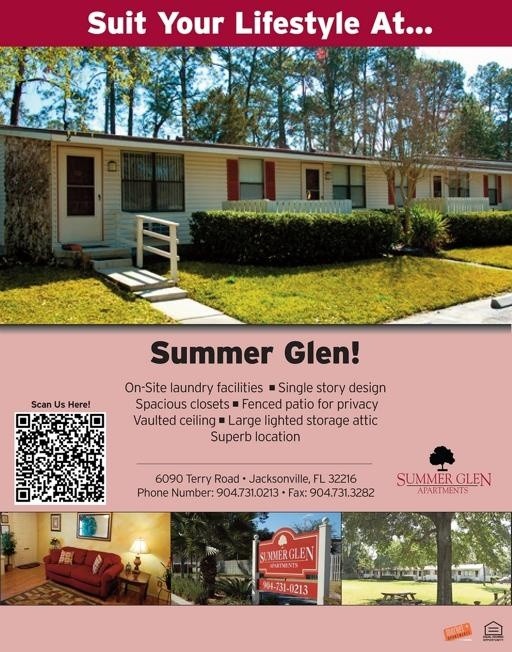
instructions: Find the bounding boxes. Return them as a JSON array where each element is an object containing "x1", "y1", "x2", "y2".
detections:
[
  {"x1": 50, "y1": 514, "x2": 61, "y2": 532},
  {"x1": 0, "y1": 524, "x2": 10, "y2": 535},
  {"x1": 75, "y1": 514, "x2": 112, "y2": 541},
  {"x1": 0, "y1": 514, "x2": 8, "y2": 524}
]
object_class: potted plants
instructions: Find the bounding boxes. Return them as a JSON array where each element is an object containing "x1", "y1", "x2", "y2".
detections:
[
  {"x1": 155, "y1": 555, "x2": 171, "y2": 591},
  {"x1": 0, "y1": 532, "x2": 17, "y2": 571}
]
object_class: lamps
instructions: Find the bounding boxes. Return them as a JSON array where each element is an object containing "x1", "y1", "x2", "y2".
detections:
[{"x1": 128, "y1": 538, "x2": 150, "y2": 575}]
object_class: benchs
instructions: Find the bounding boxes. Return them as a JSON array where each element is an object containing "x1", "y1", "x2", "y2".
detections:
[{"x1": 377, "y1": 599, "x2": 421, "y2": 606}]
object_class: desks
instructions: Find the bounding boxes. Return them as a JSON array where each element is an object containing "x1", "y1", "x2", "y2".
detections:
[
  {"x1": 381, "y1": 593, "x2": 415, "y2": 601},
  {"x1": 118, "y1": 565, "x2": 151, "y2": 605}
]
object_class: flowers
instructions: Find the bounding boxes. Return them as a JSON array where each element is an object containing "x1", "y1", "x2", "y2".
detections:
[{"x1": 50, "y1": 537, "x2": 60, "y2": 545}]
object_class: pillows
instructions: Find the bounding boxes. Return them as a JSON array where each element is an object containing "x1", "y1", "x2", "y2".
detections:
[
  {"x1": 58, "y1": 549, "x2": 74, "y2": 565},
  {"x1": 92, "y1": 554, "x2": 103, "y2": 575}
]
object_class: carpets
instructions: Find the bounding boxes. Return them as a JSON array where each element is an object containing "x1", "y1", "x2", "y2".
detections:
[
  {"x1": 15, "y1": 559, "x2": 40, "y2": 569},
  {"x1": 62, "y1": 245, "x2": 110, "y2": 250},
  {"x1": 0, "y1": 579, "x2": 106, "y2": 605}
]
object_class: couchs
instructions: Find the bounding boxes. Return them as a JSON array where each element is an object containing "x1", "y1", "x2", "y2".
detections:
[{"x1": 42, "y1": 544, "x2": 124, "y2": 601}]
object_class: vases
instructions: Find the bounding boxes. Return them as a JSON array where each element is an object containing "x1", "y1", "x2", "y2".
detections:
[{"x1": 53, "y1": 545, "x2": 57, "y2": 549}]
